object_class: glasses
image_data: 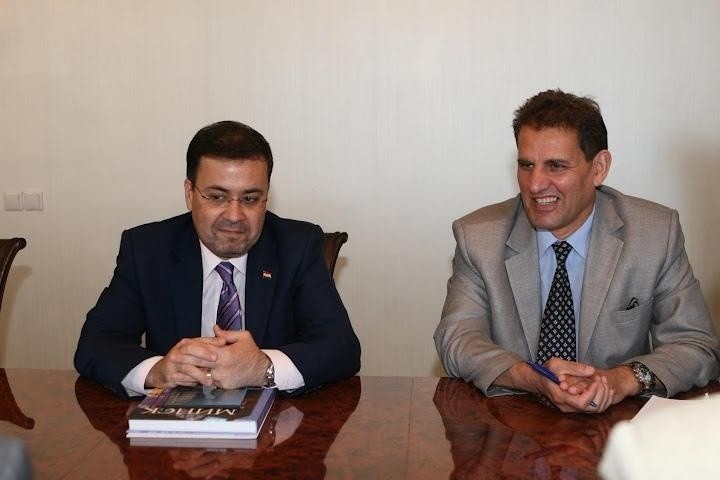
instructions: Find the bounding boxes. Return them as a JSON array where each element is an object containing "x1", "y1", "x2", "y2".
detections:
[{"x1": 192, "y1": 183, "x2": 268, "y2": 207}]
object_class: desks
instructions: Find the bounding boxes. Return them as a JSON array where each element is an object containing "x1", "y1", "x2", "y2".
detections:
[{"x1": 1, "y1": 368, "x2": 720, "y2": 480}]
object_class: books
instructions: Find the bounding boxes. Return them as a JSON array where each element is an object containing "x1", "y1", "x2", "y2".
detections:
[
  {"x1": 125, "y1": 388, "x2": 276, "y2": 440},
  {"x1": 129, "y1": 414, "x2": 276, "y2": 450}
]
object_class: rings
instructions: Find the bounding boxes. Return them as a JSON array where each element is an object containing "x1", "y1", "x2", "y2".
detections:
[{"x1": 207, "y1": 370, "x2": 212, "y2": 379}]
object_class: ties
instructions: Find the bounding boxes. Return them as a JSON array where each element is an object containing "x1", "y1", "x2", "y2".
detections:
[
  {"x1": 214, "y1": 262, "x2": 242, "y2": 338},
  {"x1": 537, "y1": 242, "x2": 577, "y2": 411}
]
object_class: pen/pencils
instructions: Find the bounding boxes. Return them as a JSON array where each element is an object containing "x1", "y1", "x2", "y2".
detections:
[{"x1": 527, "y1": 360, "x2": 598, "y2": 408}]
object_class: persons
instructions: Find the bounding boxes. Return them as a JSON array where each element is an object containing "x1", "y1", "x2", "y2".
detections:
[
  {"x1": 74, "y1": 120, "x2": 361, "y2": 400},
  {"x1": 75, "y1": 375, "x2": 361, "y2": 480},
  {"x1": 432, "y1": 377, "x2": 647, "y2": 480},
  {"x1": 433, "y1": 90, "x2": 720, "y2": 414}
]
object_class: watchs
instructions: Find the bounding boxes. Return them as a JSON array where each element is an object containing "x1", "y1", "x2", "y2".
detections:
[
  {"x1": 628, "y1": 362, "x2": 656, "y2": 394},
  {"x1": 269, "y1": 425, "x2": 278, "y2": 442},
  {"x1": 265, "y1": 364, "x2": 275, "y2": 387}
]
